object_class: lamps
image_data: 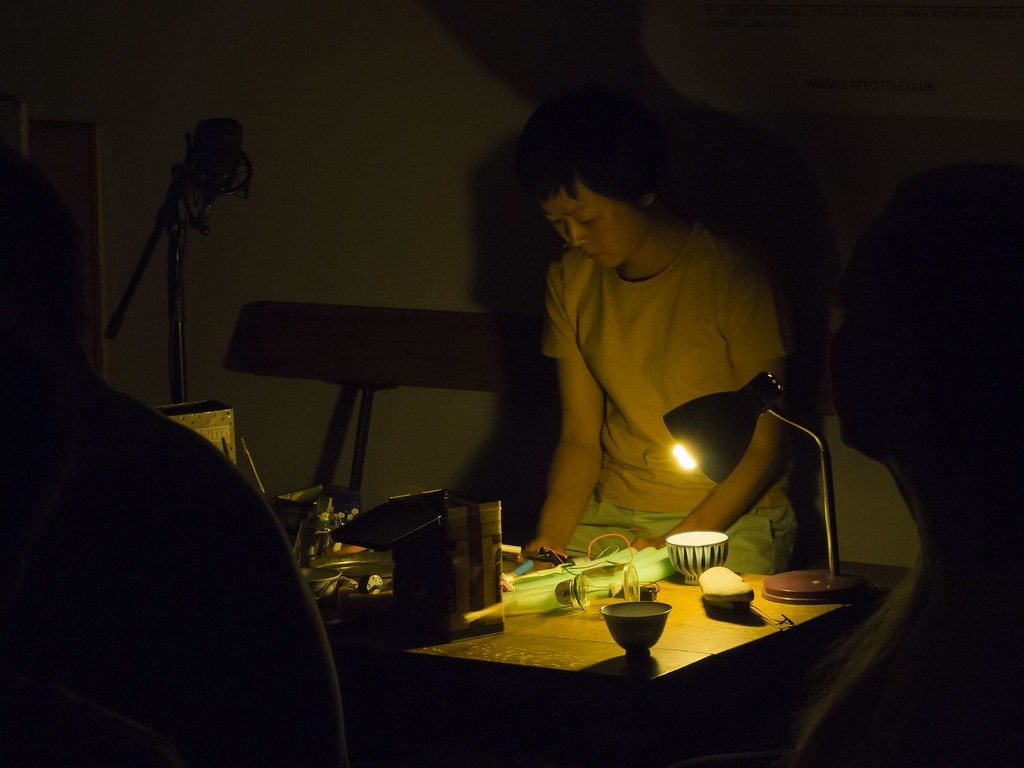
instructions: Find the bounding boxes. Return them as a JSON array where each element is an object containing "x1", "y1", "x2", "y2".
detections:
[{"x1": 664, "y1": 372, "x2": 876, "y2": 602}]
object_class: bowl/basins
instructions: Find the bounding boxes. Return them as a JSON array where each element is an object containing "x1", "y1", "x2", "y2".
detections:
[
  {"x1": 301, "y1": 568, "x2": 342, "y2": 599},
  {"x1": 665, "y1": 531, "x2": 729, "y2": 585},
  {"x1": 599, "y1": 601, "x2": 672, "y2": 661}
]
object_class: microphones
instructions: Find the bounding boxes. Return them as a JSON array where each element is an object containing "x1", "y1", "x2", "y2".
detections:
[{"x1": 188, "y1": 117, "x2": 243, "y2": 223}]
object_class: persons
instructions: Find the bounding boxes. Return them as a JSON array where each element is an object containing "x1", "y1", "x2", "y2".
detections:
[
  {"x1": 772, "y1": 167, "x2": 1024, "y2": 768},
  {"x1": 0, "y1": 136, "x2": 350, "y2": 768},
  {"x1": 502, "y1": 86, "x2": 800, "y2": 572}
]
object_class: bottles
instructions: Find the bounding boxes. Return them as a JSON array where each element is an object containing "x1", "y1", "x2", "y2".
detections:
[{"x1": 570, "y1": 563, "x2": 641, "y2": 615}]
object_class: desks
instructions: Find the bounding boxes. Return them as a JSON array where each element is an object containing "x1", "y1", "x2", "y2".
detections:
[{"x1": 326, "y1": 562, "x2": 906, "y2": 768}]
